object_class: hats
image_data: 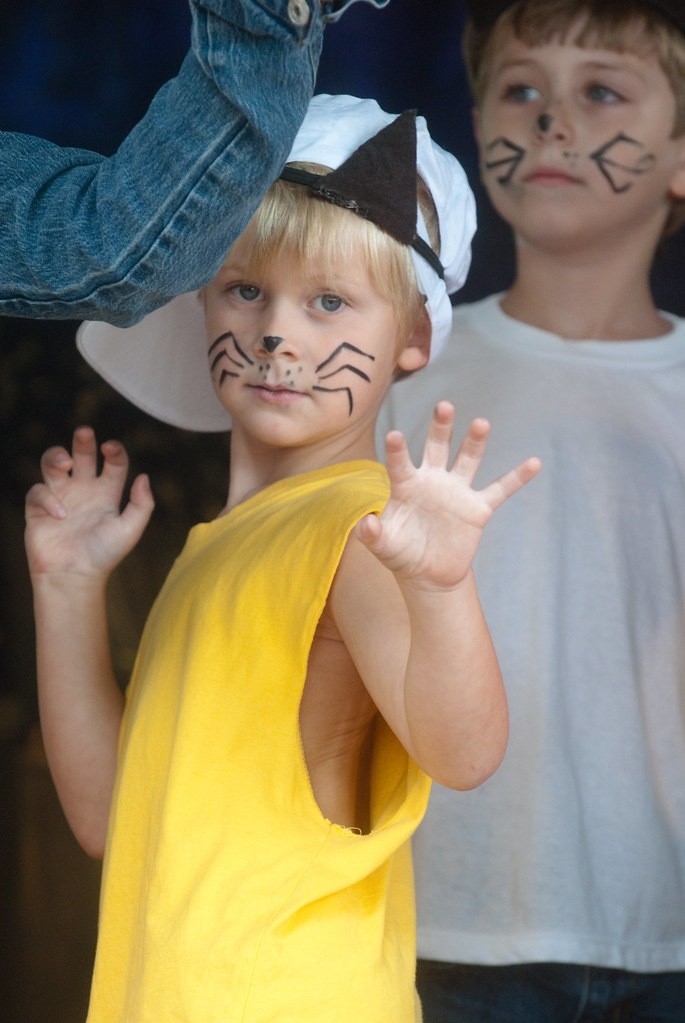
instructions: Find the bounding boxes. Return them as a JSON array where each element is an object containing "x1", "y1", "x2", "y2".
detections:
[{"x1": 75, "y1": 95, "x2": 478, "y2": 431}]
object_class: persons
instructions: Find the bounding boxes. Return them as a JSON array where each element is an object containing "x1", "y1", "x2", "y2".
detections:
[
  {"x1": 0, "y1": 0, "x2": 392, "y2": 329},
  {"x1": 25, "y1": 92, "x2": 541, "y2": 1023},
  {"x1": 376, "y1": 0, "x2": 685, "y2": 1023}
]
